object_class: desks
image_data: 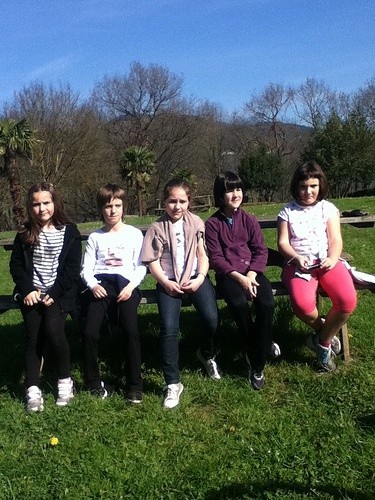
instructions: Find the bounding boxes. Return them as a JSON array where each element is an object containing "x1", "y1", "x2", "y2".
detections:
[{"x1": 0, "y1": 210, "x2": 375, "y2": 362}]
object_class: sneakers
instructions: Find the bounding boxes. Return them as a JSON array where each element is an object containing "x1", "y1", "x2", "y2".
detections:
[
  {"x1": 26, "y1": 385, "x2": 44, "y2": 412},
  {"x1": 91, "y1": 381, "x2": 107, "y2": 399},
  {"x1": 250, "y1": 365, "x2": 265, "y2": 390},
  {"x1": 315, "y1": 318, "x2": 341, "y2": 354},
  {"x1": 56, "y1": 378, "x2": 74, "y2": 406},
  {"x1": 307, "y1": 333, "x2": 337, "y2": 371},
  {"x1": 163, "y1": 382, "x2": 184, "y2": 408},
  {"x1": 269, "y1": 342, "x2": 281, "y2": 358},
  {"x1": 126, "y1": 391, "x2": 142, "y2": 403},
  {"x1": 197, "y1": 349, "x2": 220, "y2": 380}
]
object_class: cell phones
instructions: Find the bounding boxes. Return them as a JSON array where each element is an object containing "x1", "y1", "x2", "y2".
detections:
[
  {"x1": 306, "y1": 263, "x2": 322, "y2": 270},
  {"x1": 251, "y1": 285, "x2": 258, "y2": 295}
]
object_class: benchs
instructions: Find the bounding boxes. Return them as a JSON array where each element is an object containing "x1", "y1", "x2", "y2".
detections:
[{"x1": 0, "y1": 274, "x2": 375, "y2": 310}]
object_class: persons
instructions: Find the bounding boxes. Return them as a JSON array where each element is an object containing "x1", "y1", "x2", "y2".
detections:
[
  {"x1": 79, "y1": 186, "x2": 147, "y2": 403},
  {"x1": 277, "y1": 160, "x2": 357, "y2": 372},
  {"x1": 141, "y1": 178, "x2": 222, "y2": 408},
  {"x1": 9, "y1": 182, "x2": 82, "y2": 414},
  {"x1": 205, "y1": 171, "x2": 281, "y2": 390}
]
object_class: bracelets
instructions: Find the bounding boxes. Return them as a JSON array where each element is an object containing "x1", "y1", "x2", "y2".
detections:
[{"x1": 197, "y1": 273, "x2": 206, "y2": 278}]
